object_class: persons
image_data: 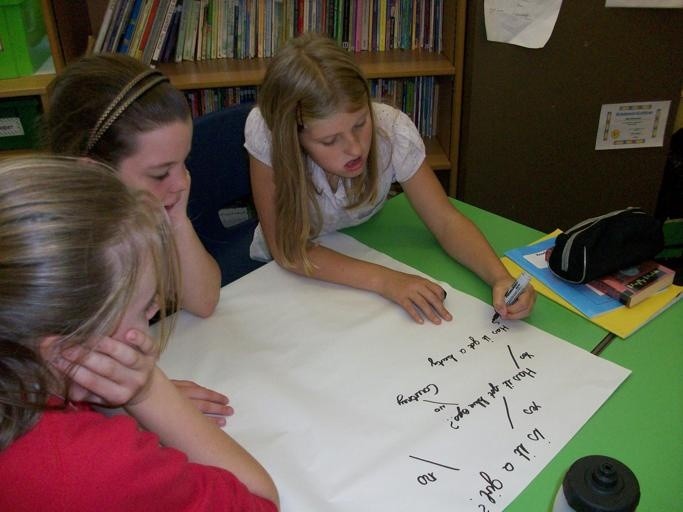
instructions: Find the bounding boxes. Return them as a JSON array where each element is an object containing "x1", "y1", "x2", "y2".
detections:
[
  {"x1": 242, "y1": 33, "x2": 535, "y2": 325},
  {"x1": 0, "y1": 154, "x2": 281, "y2": 511},
  {"x1": 45, "y1": 54, "x2": 221, "y2": 318}
]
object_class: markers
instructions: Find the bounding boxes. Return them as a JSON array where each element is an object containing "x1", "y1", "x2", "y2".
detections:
[{"x1": 492, "y1": 272, "x2": 531, "y2": 323}]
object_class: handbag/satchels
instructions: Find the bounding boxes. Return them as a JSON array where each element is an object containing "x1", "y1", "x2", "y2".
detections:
[{"x1": 547, "y1": 204, "x2": 665, "y2": 286}]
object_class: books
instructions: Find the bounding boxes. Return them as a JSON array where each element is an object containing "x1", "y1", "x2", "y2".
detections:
[
  {"x1": 363, "y1": 71, "x2": 443, "y2": 148},
  {"x1": 90, "y1": 1, "x2": 443, "y2": 60},
  {"x1": 545, "y1": 245, "x2": 676, "y2": 309},
  {"x1": 183, "y1": 85, "x2": 263, "y2": 136}
]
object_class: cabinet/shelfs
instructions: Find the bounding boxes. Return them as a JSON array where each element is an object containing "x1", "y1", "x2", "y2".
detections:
[{"x1": 0, "y1": 1, "x2": 466, "y2": 200}]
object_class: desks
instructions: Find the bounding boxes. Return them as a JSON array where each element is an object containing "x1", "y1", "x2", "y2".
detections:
[{"x1": 148, "y1": 192, "x2": 683, "y2": 512}]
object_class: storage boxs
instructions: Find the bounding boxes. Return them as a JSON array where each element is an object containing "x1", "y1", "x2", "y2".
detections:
[{"x1": 0, "y1": 100, "x2": 42, "y2": 150}]
